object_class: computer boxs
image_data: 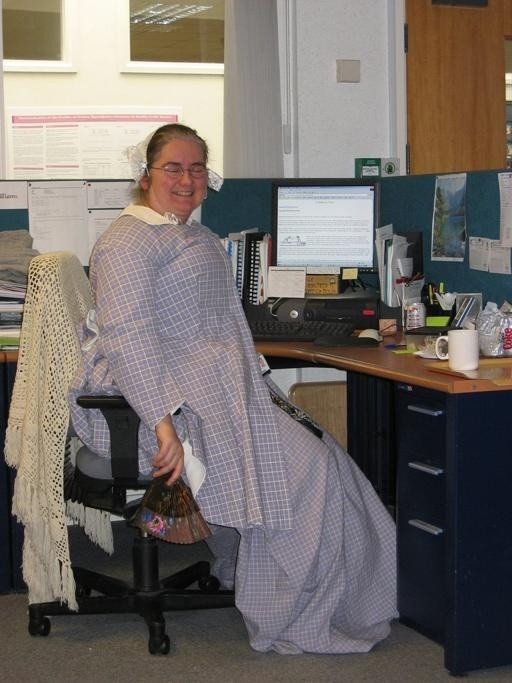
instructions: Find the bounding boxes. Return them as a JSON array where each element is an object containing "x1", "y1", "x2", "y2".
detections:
[{"x1": 264, "y1": 283, "x2": 380, "y2": 330}]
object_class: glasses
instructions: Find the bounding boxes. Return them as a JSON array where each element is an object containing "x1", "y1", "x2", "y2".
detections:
[{"x1": 153, "y1": 164, "x2": 208, "y2": 179}]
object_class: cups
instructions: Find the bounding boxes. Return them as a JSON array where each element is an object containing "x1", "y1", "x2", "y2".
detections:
[{"x1": 434, "y1": 328, "x2": 479, "y2": 370}]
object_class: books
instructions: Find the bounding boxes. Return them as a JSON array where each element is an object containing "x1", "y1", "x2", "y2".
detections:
[
  {"x1": 0, "y1": 280, "x2": 28, "y2": 351},
  {"x1": 374, "y1": 223, "x2": 414, "y2": 308},
  {"x1": 218, "y1": 227, "x2": 273, "y2": 306}
]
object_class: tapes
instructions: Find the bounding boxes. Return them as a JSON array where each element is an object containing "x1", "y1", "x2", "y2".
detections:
[{"x1": 436, "y1": 336, "x2": 448, "y2": 360}]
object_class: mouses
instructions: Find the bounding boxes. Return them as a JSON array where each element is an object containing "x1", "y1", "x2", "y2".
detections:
[{"x1": 358, "y1": 328, "x2": 383, "y2": 344}]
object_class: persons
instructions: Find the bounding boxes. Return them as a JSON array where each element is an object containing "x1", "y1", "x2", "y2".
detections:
[{"x1": 70, "y1": 124, "x2": 399, "y2": 656}]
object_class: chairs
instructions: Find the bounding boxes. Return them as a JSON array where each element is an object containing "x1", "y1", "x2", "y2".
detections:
[{"x1": 8, "y1": 250, "x2": 244, "y2": 655}]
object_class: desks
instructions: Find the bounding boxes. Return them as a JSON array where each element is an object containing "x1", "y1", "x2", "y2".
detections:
[{"x1": 1, "y1": 327, "x2": 512, "y2": 675}]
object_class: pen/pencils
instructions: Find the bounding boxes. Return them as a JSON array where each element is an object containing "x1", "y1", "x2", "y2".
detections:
[
  {"x1": 384, "y1": 344, "x2": 407, "y2": 348},
  {"x1": 426, "y1": 282, "x2": 448, "y2": 305}
]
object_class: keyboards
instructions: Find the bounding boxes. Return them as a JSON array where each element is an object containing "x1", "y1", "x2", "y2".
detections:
[{"x1": 246, "y1": 318, "x2": 355, "y2": 341}]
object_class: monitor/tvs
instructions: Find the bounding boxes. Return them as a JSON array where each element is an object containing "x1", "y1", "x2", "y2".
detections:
[{"x1": 270, "y1": 178, "x2": 379, "y2": 294}]
